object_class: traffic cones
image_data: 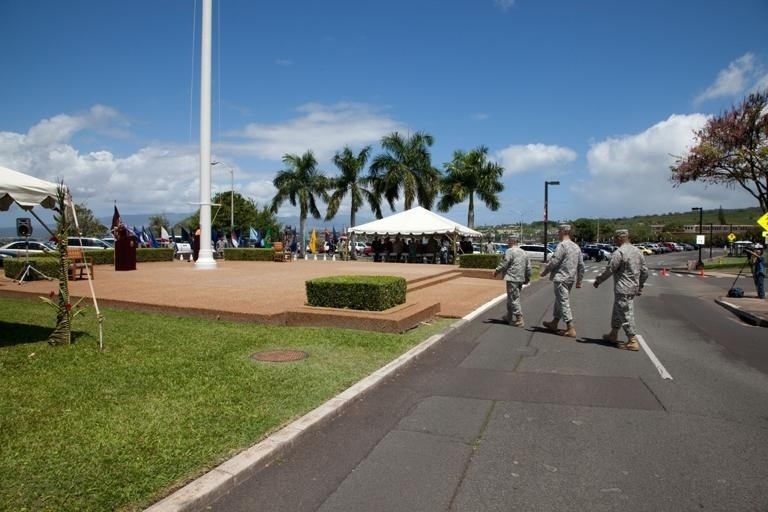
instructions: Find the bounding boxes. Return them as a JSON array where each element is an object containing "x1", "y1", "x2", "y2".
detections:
[{"x1": 661, "y1": 266, "x2": 668, "y2": 276}]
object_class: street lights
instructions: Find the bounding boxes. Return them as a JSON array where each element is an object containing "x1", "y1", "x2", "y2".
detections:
[
  {"x1": 706, "y1": 222, "x2": 712, "y2": 258},
  {"x1": 211, "y1": 161, "x2": 234, "y2": 248},
  {"x1": 692, "y1": 207, "x2": 704, "y2": 268},
  {"x1": 544, "y1": 181, "x2": 560, "y2": 262}
]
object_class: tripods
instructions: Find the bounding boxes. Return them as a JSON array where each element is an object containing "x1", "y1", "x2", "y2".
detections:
[
  {"x1": 731, "y1": 257, "x2": 759, "y2": 298},
  {"x1": 13, "y1": 236, "x2": 52, "y2": 285}
]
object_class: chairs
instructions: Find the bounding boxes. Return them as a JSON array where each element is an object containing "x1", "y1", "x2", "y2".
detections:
[
  {"x1": 67, "y1": 246, "x2": 94, "y2": 280},
  {"x1": 272, "y1": 241, "x2": 292, "y2": 263}
]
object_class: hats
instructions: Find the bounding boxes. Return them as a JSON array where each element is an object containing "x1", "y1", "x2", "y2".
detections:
[
  {"x1": 613, "y1": 229, "x2": 629, "y2": 237},
  {"x1": 557, "y1": 225, "x2": 572, "y2": 231},
  {"x1": 505, "y1": 235, "x2": 519, "y2": 242}
]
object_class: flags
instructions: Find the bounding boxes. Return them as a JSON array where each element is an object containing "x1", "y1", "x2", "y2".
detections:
[
  {"x1": 110, "y1": 205, "x2": 122, "y2": 240},
  {"x1": 333, "y1": 225, "x2": 339, "y2": 244},
  {"x1": 309, "y1": 228, "x2": 316, "y2": 255},
  {"x1": 134, "y1": 226, "x2": 195, "y2": 248},
  {"x1": 231, "y1": 230, "x2": 240, "y2": 248},
  {"x1": 249, "y1": 225, "x2": 258, "y2": 242}
]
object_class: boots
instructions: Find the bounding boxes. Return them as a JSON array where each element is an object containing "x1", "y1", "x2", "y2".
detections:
[
  {"x1": 615, "y1": 335, "x2": 641, "y2": 351},
  {"x1": 543, "y1": 317, "x2": 560, "y2": 331},
  {"x1": 602, "y1": 328, "x2": 619, "y2": 343},
  {"x1": 559, "y1": 324, "x2": 576, "y2": 338},
  {"x1": 502, "y1": 311, "x2": 524, "y2": 328}
]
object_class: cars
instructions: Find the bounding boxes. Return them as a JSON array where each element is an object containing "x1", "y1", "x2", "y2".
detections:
[
  {"x1": 335, "y1": 242, "x2": 366, "y2": 255},
  {"x1": 324, "y1": 242, "x2": 331, "y2": 252},
  {"x1": 472, "y1": 241, "x2": 699, "y2": 262},
  {"x1": 67, "y1": 237, "x2": 114, "y2": 249},
  {"x1": 0, "y1": 241, "x2": 58, "y2": 258}
]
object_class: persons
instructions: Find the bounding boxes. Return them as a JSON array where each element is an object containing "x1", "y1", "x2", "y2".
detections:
[
  {"x1": 540, "y1": 225, "x2": 585, "y2": 337},
  {"x1": 746, "y1": 248, "x2": 766, "y2": 299},
  {"x1": 166, "y1": 237, "x2": 178, "y2": 255},
  {"x1": 216, "y1": 237, "x2": 228, "y2": 259},
  {"x1": 493, "y1": 233, "x2": 531, "y2": 327},
  {"x1": 371, "y1": 236, "x2": 473, "y2": 264},
  {"x1": 593, "y1": 229, "x2": 649, "y2": 351}
]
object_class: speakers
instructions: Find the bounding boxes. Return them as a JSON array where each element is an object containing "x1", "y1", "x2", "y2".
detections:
[{"x1": 16, "y1": 217, "x2": 32, "y2": 236}]
